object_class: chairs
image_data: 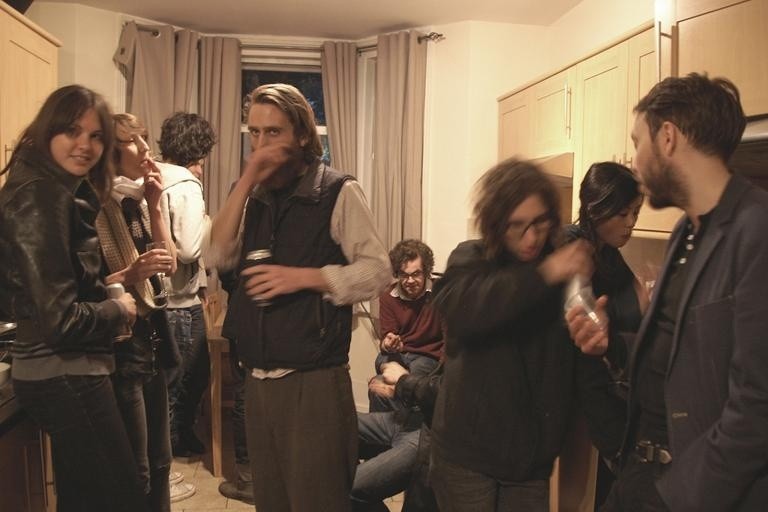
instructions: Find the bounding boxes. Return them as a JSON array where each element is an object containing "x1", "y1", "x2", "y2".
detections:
[{"x1": 201, "y1": 293, "x2": 242, "y2": 412}]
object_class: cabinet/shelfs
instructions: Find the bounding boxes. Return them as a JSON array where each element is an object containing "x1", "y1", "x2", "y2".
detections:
[
  {"x1": 0, "y1": 0, "x2": 62, "y2": 189},
  {"x1": 498, "y1": 61, "x2": 572, "y2": 188},
  {"x1": 0, "y1": 384, "x2": 59, "y2": 511},
  {"x1": 652, "y1": 1, "x2": 767, "y2": 178},
  {"x1": 571, "y1": 20, "x2": 697, "y2": 245}
]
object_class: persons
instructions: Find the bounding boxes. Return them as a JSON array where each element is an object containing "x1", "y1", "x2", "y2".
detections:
[
  {"x1": 564, "y1": 71, "x2": 768, "y2": 511},
  {"x1": 96, "y1": 112, "x2": 177, "y2": 511},
  {"x1": 366, "y1": 236, "x2": 450, "y2": 412},
  {"x1": 202, "y1": 81, "x2": 394, "y2": 511},
  {"x1": 548, "y1": 160, "x2": 649, "y2": 512},
  {"x1": 217, "y1": 177, "x2": 254, "y2": 504},
  {"x1": 347, "y1": 358, "x2": 447, "y2": 511},
  {"x1": 0, "y1": 83, "x2": 151, "y2": 512},
  {"x1": 427, "y1": 155, "x2": 598, "y2": 511},
  {"x1": 149, "y1": 111, "x2": 213, "y2": 462}
]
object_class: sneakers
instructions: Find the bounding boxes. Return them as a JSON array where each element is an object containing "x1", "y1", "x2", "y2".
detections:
[
  {"x1": 168, "y1": 482, "x2": 197, "y2": 502},
  {"x1": 169, "y1": 471, "x2": 185, "y2": 486}
]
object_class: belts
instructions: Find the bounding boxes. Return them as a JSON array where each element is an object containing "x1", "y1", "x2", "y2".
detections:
[{"x1": 630, "y1": 438, "x2": 672, "y2": 464}]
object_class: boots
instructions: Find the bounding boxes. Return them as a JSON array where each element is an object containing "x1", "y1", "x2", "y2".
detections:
[{"x1": 219, "y1": 461, "x2": 255, "y2": 505}]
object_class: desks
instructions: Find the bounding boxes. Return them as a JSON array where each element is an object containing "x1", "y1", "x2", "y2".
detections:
[{"x1": 210, "y1": 305, "x2": 245, "y2": 479}]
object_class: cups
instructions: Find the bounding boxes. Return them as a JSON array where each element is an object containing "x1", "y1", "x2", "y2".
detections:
[
  {"x1": 563, "y1": 287, "x2": 612, "y2": 333},
  {"x1": 144, "y1": 240, "x2": 173, "y2": 272}
]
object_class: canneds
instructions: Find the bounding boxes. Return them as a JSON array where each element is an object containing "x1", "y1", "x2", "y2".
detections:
[
  {"x1": 245, "y1": 249, "x2": 281, "y2": 308},
  {"x1": 105, "y1": 282, "x2": 133, "y2": 344}
]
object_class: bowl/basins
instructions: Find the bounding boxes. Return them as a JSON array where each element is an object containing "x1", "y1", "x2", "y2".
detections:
[{"x1": 0, "y1": 361, "x2": 12, "y2": 386}]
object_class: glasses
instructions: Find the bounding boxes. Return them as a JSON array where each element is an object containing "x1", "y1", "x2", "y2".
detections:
[{"x1": 398, "y1": 270, "x2": 423, "y2": 278}]
